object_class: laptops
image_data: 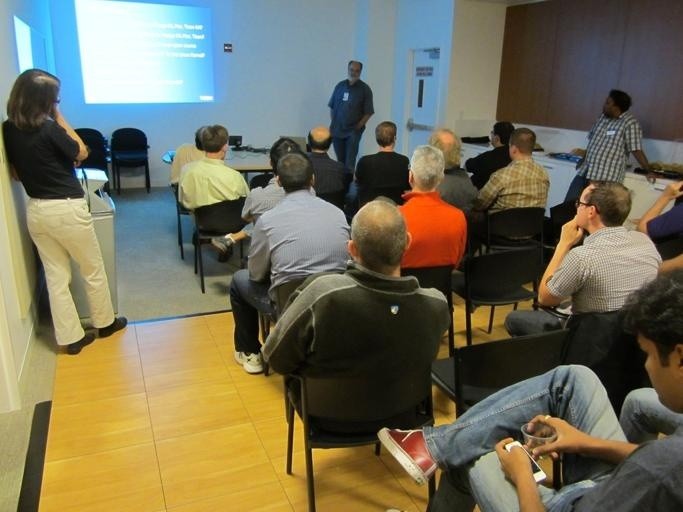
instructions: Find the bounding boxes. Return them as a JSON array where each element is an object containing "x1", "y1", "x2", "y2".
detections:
[{"x1": 278, "y1": 136, "x2": 307, "y2": 154}]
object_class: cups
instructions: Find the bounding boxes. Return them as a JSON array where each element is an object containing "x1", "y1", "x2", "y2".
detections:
[{"x1": 520, "y1": 423, "x2": 556, "y2": 460}]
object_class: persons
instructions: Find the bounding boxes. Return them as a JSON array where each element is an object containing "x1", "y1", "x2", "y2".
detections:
[{"x1": 1, "y1": 68, "x2": 128, "y2": 355}]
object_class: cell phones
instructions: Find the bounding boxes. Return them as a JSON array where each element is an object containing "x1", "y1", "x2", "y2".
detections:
[{"x1": 505, "y1": 440, "x2": 547, "y2": 484}]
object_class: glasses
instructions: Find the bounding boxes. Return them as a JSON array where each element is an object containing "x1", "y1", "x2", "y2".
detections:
[{"x1": 576, "y1": 200, "x2": 587, "y2": 208}]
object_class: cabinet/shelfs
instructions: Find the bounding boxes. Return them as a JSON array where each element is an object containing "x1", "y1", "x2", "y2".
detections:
[
  {"x1": 460, "y1": 141, "x2": 682, "y2": 232},
  {"x1": 495, "y1": 0, "x2": 682, "y2": 141}
]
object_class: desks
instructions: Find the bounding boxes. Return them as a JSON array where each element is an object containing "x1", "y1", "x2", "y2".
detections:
[{"x1": 160, "y1": 144, "x2": 275, "y2": 187}]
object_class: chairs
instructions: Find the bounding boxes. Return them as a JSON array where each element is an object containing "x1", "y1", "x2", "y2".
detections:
[
  {"x1": 451, "y1": 196, "x2": 589, "y2": 346},
  {"x1": 171, "y1": 182, "x2": 232, "y2": 260},
  {"x1": 653, "y1": 236, "x2": 682, "y2": 261},
  {"x1": 562, "y1": 310, "x2": 654, "y2": 422},
  {"x1": 192, "y1": 195, "x2": 249, "y2": 294},
  {"x1": 74, "y1": 128, "x2": 108, "y2": 194},
  {"x1": 284, "y1": 364, "x2": 436, "y2": 510},
  {"x1": 260, "y1": 277, "x2": 306, "y2": 423},
  {"x1": 431, "y1": 329, "x2": 567, "y2": 492},
  {"x1": 400, "y1": 264, "x2": 454, "y2": 358},
  {"x1": 316, "y1": 188, "x2": 346, "y2": 211},
  {"x1": 110, "y1": 127, "x2": 150, "y2": 197},
  {"x1": 249, "y1": 174, "x2": 275, "y2": 191},
  {"x1": 358, "y1": 186, "x2": 412, "y2": 208}
]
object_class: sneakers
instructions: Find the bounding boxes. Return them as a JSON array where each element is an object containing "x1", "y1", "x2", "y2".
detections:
[
  {"x1": 98, "y1": 317, "x2": 127, "y2": 337},
  {"x1": 68, "y1": 333, "x2": 95, "y2": 354},
  {"x1": 211, "y1": 236, "x2": 233, "y2": 254},
  {"x1": 234, "y1": 351, "x2": 263, "y2": 373},
  {"x1": 378, "y1": 428, "x2": 438, "y2": 485}
]
